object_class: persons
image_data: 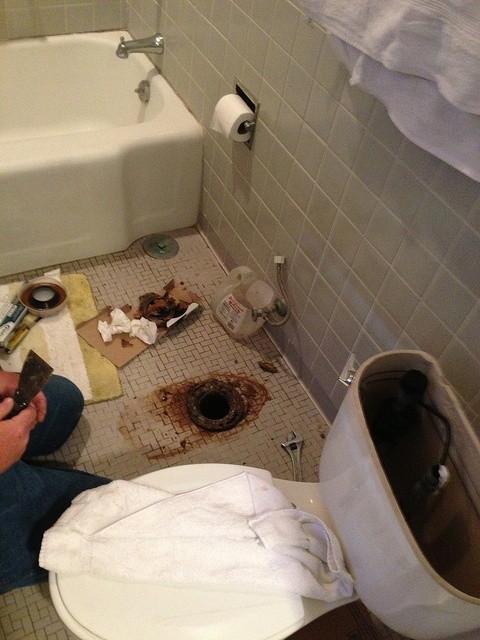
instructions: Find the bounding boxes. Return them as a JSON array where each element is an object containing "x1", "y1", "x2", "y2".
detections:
[{"x1": 1, "y1": 353, "x2": 132, "y2": 599}]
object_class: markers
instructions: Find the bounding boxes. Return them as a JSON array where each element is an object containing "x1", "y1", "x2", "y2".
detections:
[{"x1": 0, "y1": 304, "x2": 18, "y2": 340}]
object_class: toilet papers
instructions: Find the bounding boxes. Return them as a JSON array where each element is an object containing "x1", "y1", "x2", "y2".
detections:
[{"x1": 210, "y1": 94, "x2": 255, "y2": 143}]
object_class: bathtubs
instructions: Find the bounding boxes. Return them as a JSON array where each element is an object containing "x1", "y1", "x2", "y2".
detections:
[{"x1": 1, "y1": 30, "x2": 203, "y2": 277}]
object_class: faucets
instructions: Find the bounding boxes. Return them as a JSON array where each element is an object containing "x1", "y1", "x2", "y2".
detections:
[{"x1": 116, "y1": 31, "x2": 165, "y2": 59}]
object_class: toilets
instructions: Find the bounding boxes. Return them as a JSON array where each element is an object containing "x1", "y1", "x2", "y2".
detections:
[{"x1": 48, "y1": 349, "x2": 480, "y2": 635}]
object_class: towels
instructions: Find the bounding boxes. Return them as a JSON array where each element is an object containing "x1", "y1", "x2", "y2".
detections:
[
  {"x1": 39, "y1": 471, "x2": 355, "y2": 601},
  {"x1": 297, "y1": 1, "x2": 480, "y2": 182}
]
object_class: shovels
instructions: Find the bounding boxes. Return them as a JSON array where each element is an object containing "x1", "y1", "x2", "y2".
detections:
[{"x1": 8, "y1": 349, "x2": 54, "y2": 418}]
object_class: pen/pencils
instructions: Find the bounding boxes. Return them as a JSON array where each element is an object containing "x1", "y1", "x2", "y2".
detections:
[
  {"x1": 15, "y1": 308, "x2": 28, "y2": 326},
  {"x1": 11, "y1": 307, "x2": 24, "y2": 323}
]
